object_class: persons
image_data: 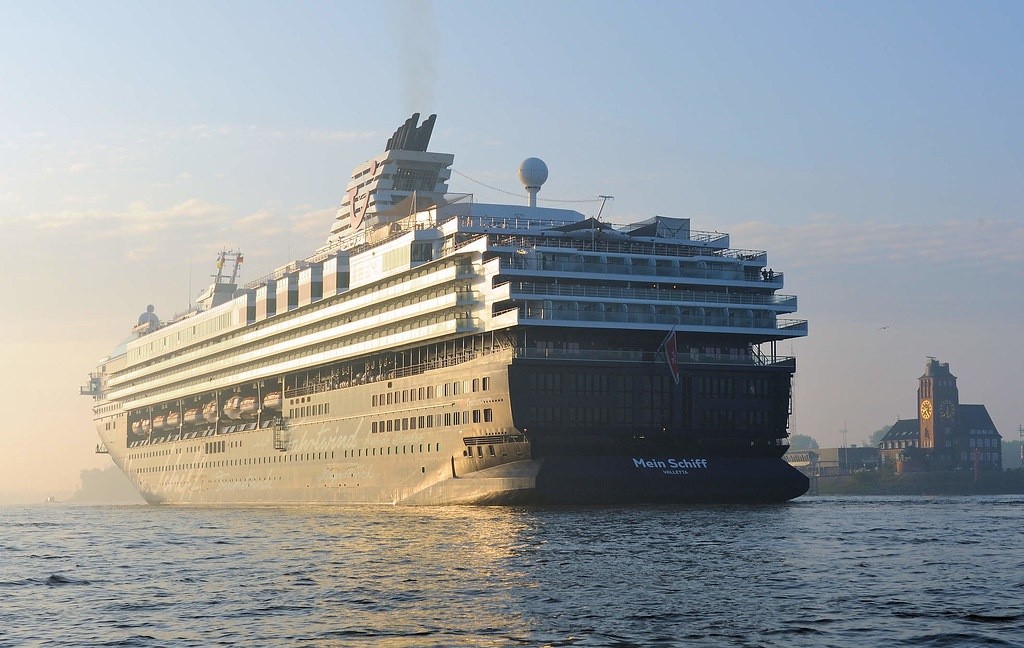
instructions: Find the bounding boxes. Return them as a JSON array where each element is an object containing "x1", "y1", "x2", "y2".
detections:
[
  {"x1": 762, "y1": 269, "x2": 768, "y2": 282},
  {"x1": 768, "y1": 268, "x2": 774, "y2": 282}
]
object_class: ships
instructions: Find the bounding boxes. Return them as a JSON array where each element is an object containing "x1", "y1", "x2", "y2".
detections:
[{"x1": 79, "y1": 112, "x2": 811, "y2": 512}]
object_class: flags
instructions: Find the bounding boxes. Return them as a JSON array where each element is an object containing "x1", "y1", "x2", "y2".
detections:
[{"x1": 662, "y1": 330, "x2": 679, "y2": 385}]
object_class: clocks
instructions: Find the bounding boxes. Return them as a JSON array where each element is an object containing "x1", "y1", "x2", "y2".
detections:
[
  {"x1": 937, "y1": 399, "x2": 956, "y2": 421},
  {"x1": 920, "y1": 399, "x2": 933, "y2": 420}
]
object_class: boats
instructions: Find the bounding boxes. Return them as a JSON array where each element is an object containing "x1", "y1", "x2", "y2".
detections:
[{"x1": 130, "y1": 391, "x2": 282, "y2": 435}]
